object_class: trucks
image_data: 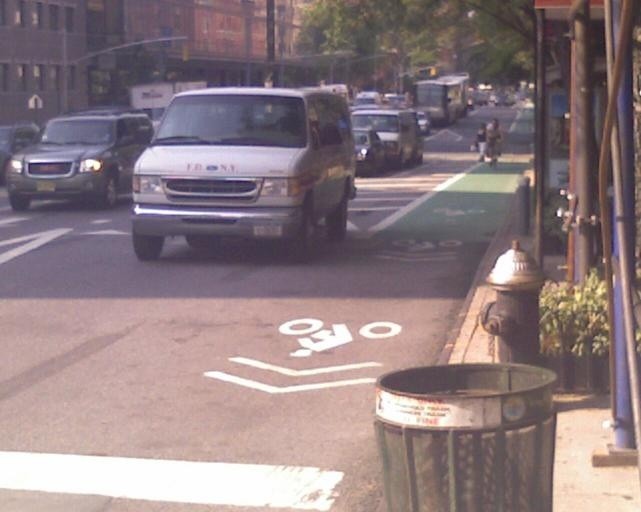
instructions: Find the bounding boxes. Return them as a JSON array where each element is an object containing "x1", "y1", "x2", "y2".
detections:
[{"x1": 128, "y1": 80, "x2": 208, "y2": 140}]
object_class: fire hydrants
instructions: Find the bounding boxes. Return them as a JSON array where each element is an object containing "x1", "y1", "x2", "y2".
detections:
[{"x1": 481, "y1": 242, "x2": 541, "y2": 367}]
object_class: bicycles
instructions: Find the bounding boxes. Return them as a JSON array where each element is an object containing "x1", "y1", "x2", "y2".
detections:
[{"x1": 487, "y1": 136, "x2": 499, "y2": 168}]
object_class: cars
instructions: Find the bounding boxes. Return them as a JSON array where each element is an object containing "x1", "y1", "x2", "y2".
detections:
[
  {"x1": 466, "y1": 81, "x2": 525, "y2": 105},
  {"x1": 342, "y1": 90, "x2": 430, "y2": 170}
]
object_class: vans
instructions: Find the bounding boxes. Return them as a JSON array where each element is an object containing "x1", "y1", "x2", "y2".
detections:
[{"x1": 131, "y1": 87, "x2": 355, "y2": 261}]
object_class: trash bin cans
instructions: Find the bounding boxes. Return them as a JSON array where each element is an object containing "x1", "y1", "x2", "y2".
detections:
[{"x1": 373, "y1": 363, "x2": 558, "y2": 512}]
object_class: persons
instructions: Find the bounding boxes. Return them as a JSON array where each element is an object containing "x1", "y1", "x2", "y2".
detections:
[
  {"x1": 486, "y1": 119, "x2": 504, "y2": 169},
  {"x1": 474, "y1": 121, "x2": 487, "y2": 163}
]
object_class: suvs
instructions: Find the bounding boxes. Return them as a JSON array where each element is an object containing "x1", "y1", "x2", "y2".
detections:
[{"x1": 2, "y1": 110, "x2": 154, "y2": 210}]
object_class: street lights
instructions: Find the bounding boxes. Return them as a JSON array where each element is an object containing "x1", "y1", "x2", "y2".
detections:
[{"x1": 63, "y1": 35, "x2": 190, "y2": 106}]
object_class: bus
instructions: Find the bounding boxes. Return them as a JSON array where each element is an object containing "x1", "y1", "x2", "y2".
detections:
[{"x1": 411, "y1": 72, "x2": 469, "y2": 126}]
